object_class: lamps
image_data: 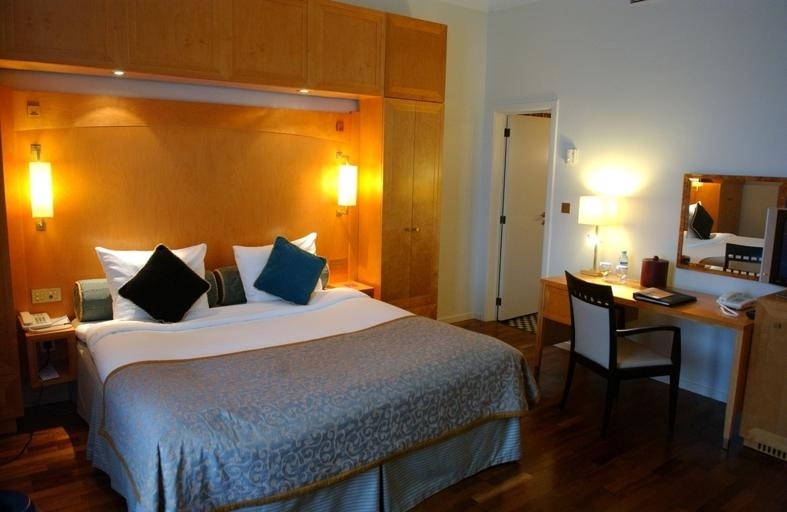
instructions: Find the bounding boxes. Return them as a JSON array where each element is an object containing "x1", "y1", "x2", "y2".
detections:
[
  {"x1": 27, "y1": 144, "x2": 55, "y2": 232},
  {"x1": 337, "y1": 151, "x2": 359, "y2": 219},
  {"x1": 578, "y1": 198, "x2": 612, "y2": 278}
]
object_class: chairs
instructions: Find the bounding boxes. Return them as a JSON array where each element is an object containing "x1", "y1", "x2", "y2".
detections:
[
  {"x1": 724, "y1": 242, "x2": 763, "y2": 276},
  {"x1": 558, "y1": 269, "x2": 682, "y2": 441}
]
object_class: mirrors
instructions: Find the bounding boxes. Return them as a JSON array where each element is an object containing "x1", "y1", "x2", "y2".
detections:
[{"x1": 675, "y1": 175, "x2": 787, "y2": 281}]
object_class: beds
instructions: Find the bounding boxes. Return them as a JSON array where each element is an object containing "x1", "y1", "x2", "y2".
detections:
[
  {"x1": 71, "y1": 287, "x2": 539, "y2": 512},
  {"x1": 682, "y1": 229, "x2": 764, "y2": 279}
]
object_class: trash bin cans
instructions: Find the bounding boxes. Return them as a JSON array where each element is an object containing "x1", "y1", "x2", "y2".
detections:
[{"x1": 0, "y1": 490, "x2": 35, "y2": 512}]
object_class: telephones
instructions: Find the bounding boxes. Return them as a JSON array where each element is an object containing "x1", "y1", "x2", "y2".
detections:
[
  {"x1": 717, "y1": 291, "x2": 754, "y2": 311},
  {"x1": 18, "y1": 312, "x2": 52, "y2": 331}
]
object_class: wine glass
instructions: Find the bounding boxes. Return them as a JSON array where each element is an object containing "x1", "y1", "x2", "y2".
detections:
[{"x1": 599, "y1": 261, "x2": 611, "y2": 282}]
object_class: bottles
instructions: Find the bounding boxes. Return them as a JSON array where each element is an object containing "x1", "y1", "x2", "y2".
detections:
[{"x1": 619, "y1": 250, "x2": 629, "y2": 283}]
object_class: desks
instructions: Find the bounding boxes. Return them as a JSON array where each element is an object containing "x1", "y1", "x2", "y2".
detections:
[{"x1": 531, "y1": 271, "x2": 755, "y2": 449}]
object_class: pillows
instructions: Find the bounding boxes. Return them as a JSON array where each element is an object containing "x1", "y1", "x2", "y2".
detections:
[
  {"x1": 684, "y1": 202, "x2": 713, "y2": 239},
  {"x1": 72, "y1": 231, "x2": 330, "y2": 323}
]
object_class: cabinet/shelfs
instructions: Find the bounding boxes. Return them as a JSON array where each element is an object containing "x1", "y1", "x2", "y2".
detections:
[
  {"x1": 355, "y1": 97, "x2": 439, "y2": 318},
  {"x1": 736, "y1": 292, "x2": 785, "y2": 458},
  {"x1": 1, "y1": 0, "x2": 444, "y2": 112},
  {"x1": 329, "y1": 279, "x2": 377, "y2": 299},
  {"x1": 25, "y1": 326, "x2": 78, "y2": 387}
]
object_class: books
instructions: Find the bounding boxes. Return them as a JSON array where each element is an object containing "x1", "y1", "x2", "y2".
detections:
[{"x1": 633, "y1": 287, "x2": 697, "y2": 307}]
